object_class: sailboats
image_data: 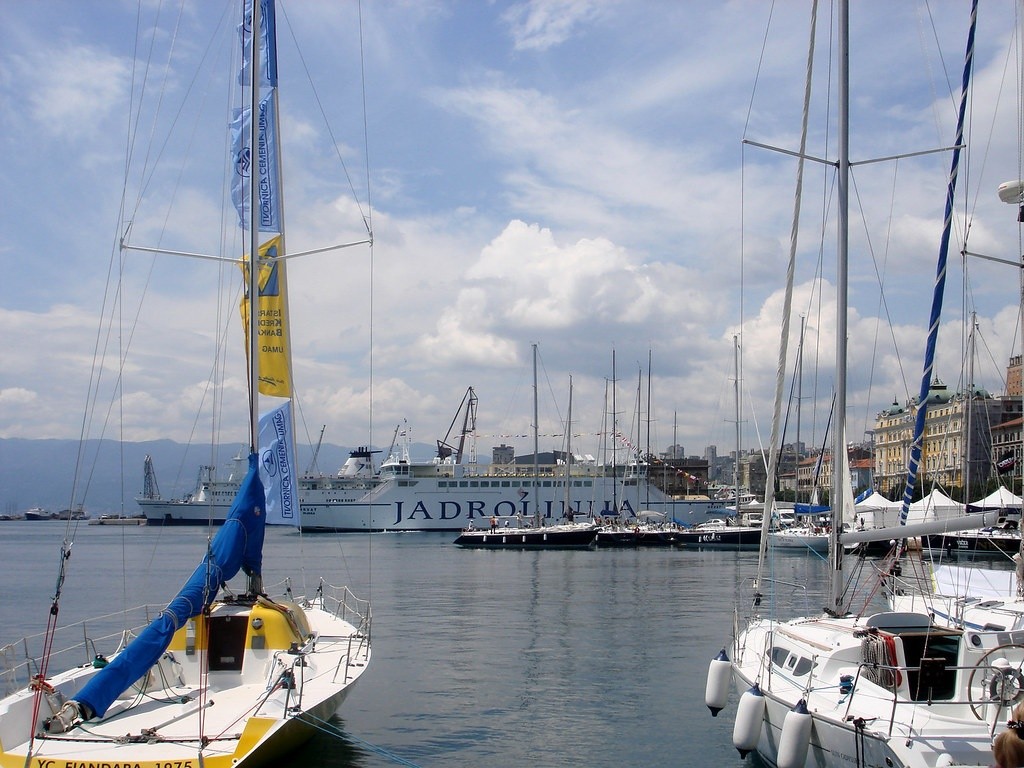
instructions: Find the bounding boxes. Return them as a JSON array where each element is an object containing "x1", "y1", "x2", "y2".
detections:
[
  {"x1": 502, "y1": 343, "x2": 675, "y2": 532},
  {"x1": 0, "y1": 0, "x2": 377, "y2": 768},
  {"x1": 727, "y1": 0, "x2": 1024, "y2": 768},
  {"x1": 918, "y1": 308, "x2": 1021, "y2": 557},
  {"x1": 767, "y1": 316, "x2": 830, "y2": 551}
]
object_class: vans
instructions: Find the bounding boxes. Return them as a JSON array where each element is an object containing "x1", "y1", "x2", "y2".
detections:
[{"x1": 743, "y1": 513, "x2": 763, "y2": 527}]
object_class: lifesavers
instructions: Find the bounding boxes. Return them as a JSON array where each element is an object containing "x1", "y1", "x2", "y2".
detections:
[{"x1": 604, "y1": 518, "x2": 611, "y2": 527}]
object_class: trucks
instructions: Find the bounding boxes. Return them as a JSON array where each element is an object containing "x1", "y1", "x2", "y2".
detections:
[{"x1": 777, "y1": 509, "x2": 796, "y2": 528}]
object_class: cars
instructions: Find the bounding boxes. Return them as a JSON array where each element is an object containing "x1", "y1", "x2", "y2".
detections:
[
  {"x1": 102, "y1": 514, "x2": 108, "y2": 518},
  {"x1": 698, "y1": 519, "x2": 725, "y2": 527}
]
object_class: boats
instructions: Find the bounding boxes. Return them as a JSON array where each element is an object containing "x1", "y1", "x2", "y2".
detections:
[
  {"x1": 834, "y1": 507, "x2": 897, "y2": 553},
  {"x1": 25, "y1": 507, "x2": 52, "y2": 520},
  {"x1": 133, "y1": 384, "x2": 379, "y2": 524},
  {"x1": 288, "y1": 386, "x2": 740, "y2": 534}
]
